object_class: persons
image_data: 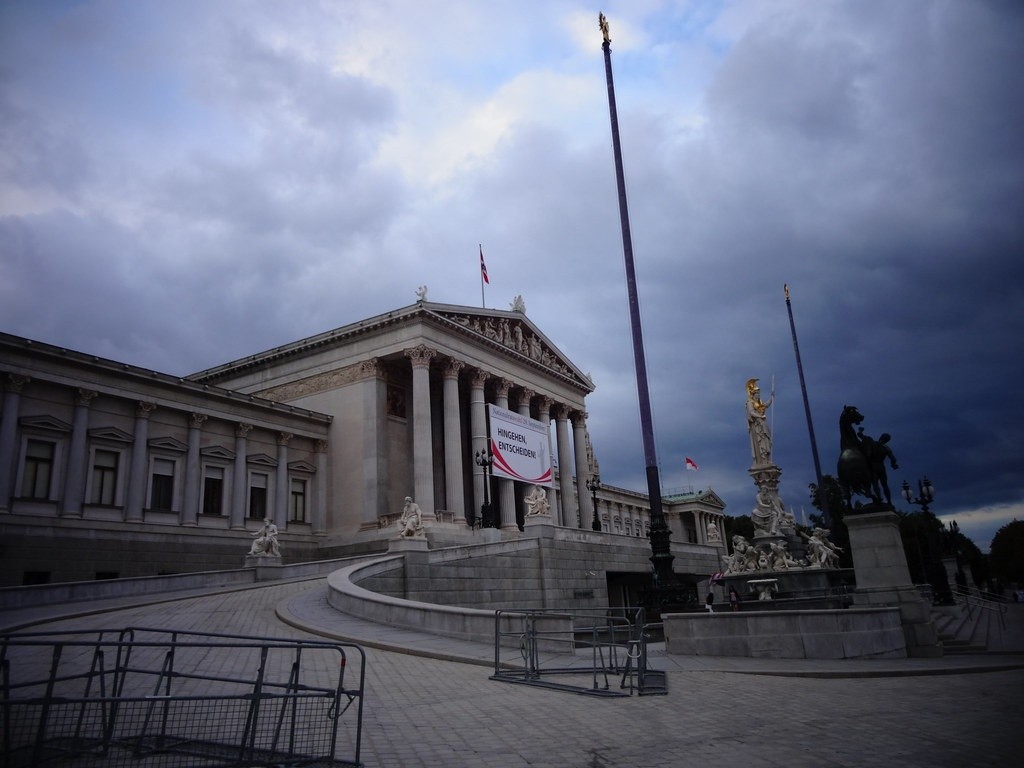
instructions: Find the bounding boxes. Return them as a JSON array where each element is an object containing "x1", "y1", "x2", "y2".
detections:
[
  {"x1": 451, "y1": 312, "x2": 577, "y2": 379},
  {"x1": 728, "y1": 585, "x2": 742, "y2": 611},
  {"x1": 745, "y1": 377, "x2": 775, "y2": 467},
  {"x1": 706, "y1": 484, "x2": 846, "y2": 600},
  {"x1": 857, "y1": 426, "x2": 901, "y2": 507},
  {"x1": 524, "y1": 483, "x2": 551, "y2": 516},
  {"x1": 397, "y1": 495, "x2": 422, "y2": 539},
  {"x1": 247, "y1": 517, "x2": 282, "y2": 558}
]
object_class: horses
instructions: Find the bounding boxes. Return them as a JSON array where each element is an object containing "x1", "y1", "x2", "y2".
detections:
[{"x1": 838, "y1": 404, "x2": 880, "y2": 510}]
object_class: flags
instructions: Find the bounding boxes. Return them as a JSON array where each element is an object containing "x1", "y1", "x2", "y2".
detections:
[
  {"x1": 480, "y1": 248, "x2": 490, "y2": 285},
  {"x1": 686, "y1": 456, "x2": 700, "y2": 471}
]
